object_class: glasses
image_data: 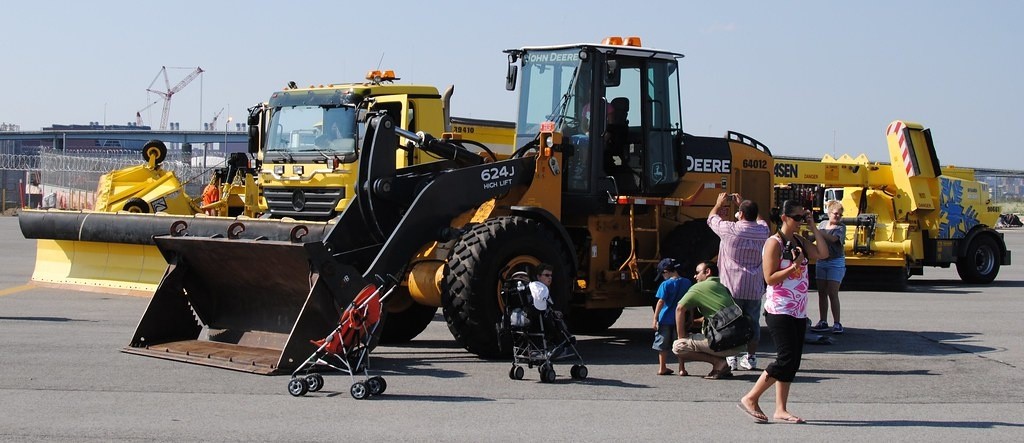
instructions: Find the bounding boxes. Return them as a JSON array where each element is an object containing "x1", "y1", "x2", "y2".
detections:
[
  {"x1": 830, "y1": 212, "x2": 843, "y2": 217},
  {"x1": 787, "y1": 213, "x2": 807, "y2": 222},
  {"x1": 540, "y1": 274, "x2": 552, "y2": 277},
  {"x1": 695, "y1": 269, "x2": 706, "y2": 275}
]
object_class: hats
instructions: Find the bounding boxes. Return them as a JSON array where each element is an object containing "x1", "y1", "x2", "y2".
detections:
[{"x1": 655, "y1": 256, "x2": 677, "y2": 281}]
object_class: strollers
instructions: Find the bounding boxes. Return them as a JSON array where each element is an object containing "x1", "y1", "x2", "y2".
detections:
[
  {"x1": 288, "y1": 273, "x2": 398, "y2": 401},
  {"x1": 500, "y1": 274, "x2": 588, "y2": 383}
]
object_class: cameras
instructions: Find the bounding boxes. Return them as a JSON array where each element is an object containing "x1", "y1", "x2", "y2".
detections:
[
  {"x1": 727, "y1": 195, "x2": 734, "y2": 201},
  {"x1": 783, "y1": 242, "x2": 800, "y2": 261}
]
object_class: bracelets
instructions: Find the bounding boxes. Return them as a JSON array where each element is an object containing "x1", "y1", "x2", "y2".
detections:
[{"x1": 792, "y1": 263, "x2": 799, "y2": 271}]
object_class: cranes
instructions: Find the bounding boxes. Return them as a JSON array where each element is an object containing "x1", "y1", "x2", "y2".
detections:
[{"x1": 136, "y1": 66, "x2": 204, "y2": 131}]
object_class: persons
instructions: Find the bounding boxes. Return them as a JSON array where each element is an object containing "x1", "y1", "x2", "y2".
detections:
[
  {"x1": 653, "y1": 258, "x2": 694, "y2": 376},
  {"x1": 571, "y1": 84, "x2": 614, "y2": 180},
  {"x1": 672, "y1": 260, "x2": 748, "y2": 380},
  {"x1": 737, "y1": 199, "x2": 830, "y2": 424},
  {"x1": 802, "y1": 200, "x2": 846, "y2": 334},
  {"x1": 528, "y1": 263, "x2": 570, "y2": 359},
  {"x1": 707, "y1": 192, "x2": 770, "y2": 371},
  {"x1": 202, "y1": 173, "x2": 222, "y2": 216}
]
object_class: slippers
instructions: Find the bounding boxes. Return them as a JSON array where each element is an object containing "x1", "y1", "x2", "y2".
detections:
[
  {"x1": 659, "y1": 369, "x2": 674, "y2": 375},
  {"x1": 773, "y1": 414, "x2": 806, "y2": 424},
  {"x1": 679, "y1": 370, "x2": 687, "y2": 376},
  {"x1": 737, "y1": 397, "x2": 768, "y2": 422}
]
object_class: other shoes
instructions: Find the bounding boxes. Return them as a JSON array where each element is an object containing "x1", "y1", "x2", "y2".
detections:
[{"x1": 705, "y1": 366, "x2": 733, "y2": 378}]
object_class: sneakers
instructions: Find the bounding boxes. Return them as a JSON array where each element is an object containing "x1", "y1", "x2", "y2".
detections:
[
  {"x1": 728, "y1": 356, "x2": 737, "y2": 370},
  {"x1": 832, "y1": 322, "x2": 843, "y2": 332},
  {"x1": 740, "y1": 355, "x2": 756, "y2": 370},
  {"x1": 811, "y1": 320, "x2": 830, "y2": 331}
]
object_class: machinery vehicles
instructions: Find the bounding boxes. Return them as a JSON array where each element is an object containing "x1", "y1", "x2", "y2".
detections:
[
  {"x1": 774, "y1": 120, "x2": 1011, "y2": 291},
  {"x1": 118, "y1": 38, "x2": 775, "y2": 375},
  {"x1": 19, "y1": 71, "x2": 517, "y2": 299}
]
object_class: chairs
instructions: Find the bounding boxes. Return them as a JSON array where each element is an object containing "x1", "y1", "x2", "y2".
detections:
[{"x1": 604, "y1": 96, "x2": 632, "y2": 161}]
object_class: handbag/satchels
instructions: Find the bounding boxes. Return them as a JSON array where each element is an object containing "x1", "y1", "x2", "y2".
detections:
[{"x1": 705, "y1": 303, "x2": 755, "y2": 352}]
